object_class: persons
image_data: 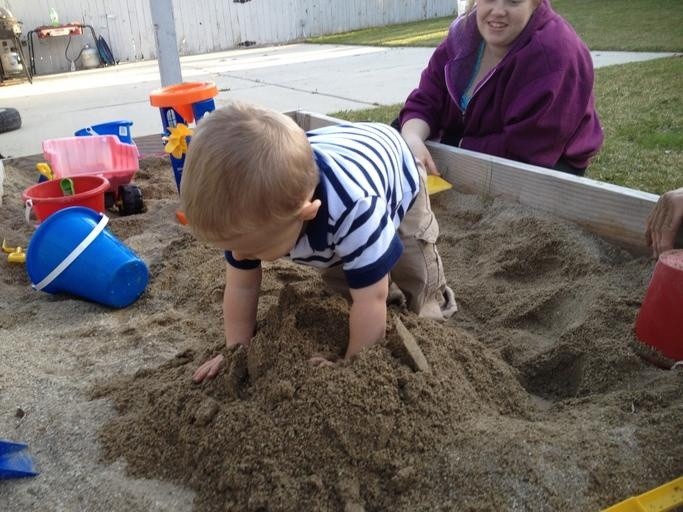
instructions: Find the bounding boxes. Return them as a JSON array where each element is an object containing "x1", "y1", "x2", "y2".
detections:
[
  {"x1": 179, "y1": 101, "x2": 459, "y2": 385},
  {"x1": 390, "y1": 0, "x2": 604, "y2": 177},
  {"x1": 644, "y1": 187, "x2": 683, "y2": 259}
]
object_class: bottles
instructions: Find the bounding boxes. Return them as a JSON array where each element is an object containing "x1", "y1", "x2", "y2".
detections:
[
  {"x1": 70, "y1": 61, "x2": 76, "y2": 72},
  {"x1": 49, "y1": 8, "x2": 59, "y2": 27}
]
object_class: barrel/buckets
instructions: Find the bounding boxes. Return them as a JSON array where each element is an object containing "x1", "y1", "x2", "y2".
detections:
[
  {"x1": 23, "y1": 174, "x2": 112, "y2": 224},
  {"x1": 637, "y1": 249, "x2": 683, "y2": 368},
  {"x1": 74, "y1": 120, "x2": 134, "y2": 143},
  {"x1": 27, "y1": 205, "x2": 149, "y2": 308}
]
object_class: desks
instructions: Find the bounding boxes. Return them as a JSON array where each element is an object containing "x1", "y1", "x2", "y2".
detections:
[{"x1": 0, "y1": 30, "x2": 33, "y2": 84}]
